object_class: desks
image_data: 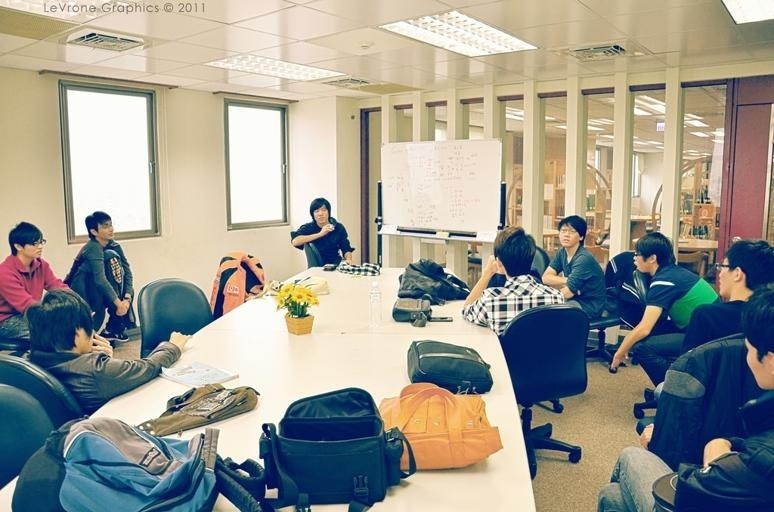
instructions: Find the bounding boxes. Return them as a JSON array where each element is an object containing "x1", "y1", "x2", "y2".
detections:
[
  {"x1": 543, "y1": 213, "x2": 718, "y2": 251},
  {"x1": 0, "y1": 267, "x2": 536, "y2": 512}
]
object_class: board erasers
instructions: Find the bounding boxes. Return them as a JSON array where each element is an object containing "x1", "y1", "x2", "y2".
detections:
[{"x1": 435, "y1": 231, "x2": 451, "y2": 237}]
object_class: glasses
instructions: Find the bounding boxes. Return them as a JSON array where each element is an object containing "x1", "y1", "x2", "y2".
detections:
[
  {"x1": 714, "y1": 262, "x2": 730, "y2": 271},
  {"x1": 32, "y1": 239, "x2": 47, "y2": 247}
]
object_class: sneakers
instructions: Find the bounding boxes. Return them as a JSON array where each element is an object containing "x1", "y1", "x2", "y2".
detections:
[{"x1": 99, "y1": 328, "x2": 130, "y2": 342}]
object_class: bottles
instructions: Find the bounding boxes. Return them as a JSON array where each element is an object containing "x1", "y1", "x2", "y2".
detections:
[
  {"x1": 368, "y1": 282, "x2": 383, "y2": 323},
  {"x1": 693, "y1": 225, "x2": 706, "y2": 239}
]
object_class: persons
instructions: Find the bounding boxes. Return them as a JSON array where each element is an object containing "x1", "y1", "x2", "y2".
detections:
[
  {"x1": 0, "y1": 222, "x2": 75, "y2": 343},
  {"x1": 611, "y1": 231, "x2": 724, "y2": 387},
  {"x1": 26, "y1": 289, "x2": 191, "y2": 416},
  {"x1": 653, "y1": 240, "x2": 774, "y2": 402},
  {"x1": 596, "y1": 287, "x2": 774, "y2": 511},
  {"x1": 291, "y1": 198, "x2": 355, "y2": 269},
  {"x1": 540, "y1": 216, "x2": 606, "y2": 321},
  {"x1": 461, "y1": 226, "x2": 566, "y2": 336},
  {"x1": 62, "y1": 212, "x2": 134, "y2": 342}
]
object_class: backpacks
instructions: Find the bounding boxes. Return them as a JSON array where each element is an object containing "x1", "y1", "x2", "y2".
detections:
[{"x1": 11, "y1": 415, "x2": 278, "y2": 512}]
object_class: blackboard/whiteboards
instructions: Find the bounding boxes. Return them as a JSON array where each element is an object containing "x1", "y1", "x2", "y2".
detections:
[{"x1": 377, "y1": 138, "x2": 503, "y2": 242}]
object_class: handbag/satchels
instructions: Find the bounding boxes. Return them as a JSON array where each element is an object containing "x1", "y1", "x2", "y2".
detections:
[
  {"x1": 378, "y1": 377, "x2": 504, "y2": 474},
  {"x1": 260, "y1": 389, "x2": 415, "y2": 508},
  {"x1": 390, "y1": 291, "x2": 436, "y2": 324},
  {"x1": 402, "y1": 330, "x2": 497, "y2": 395}
]
